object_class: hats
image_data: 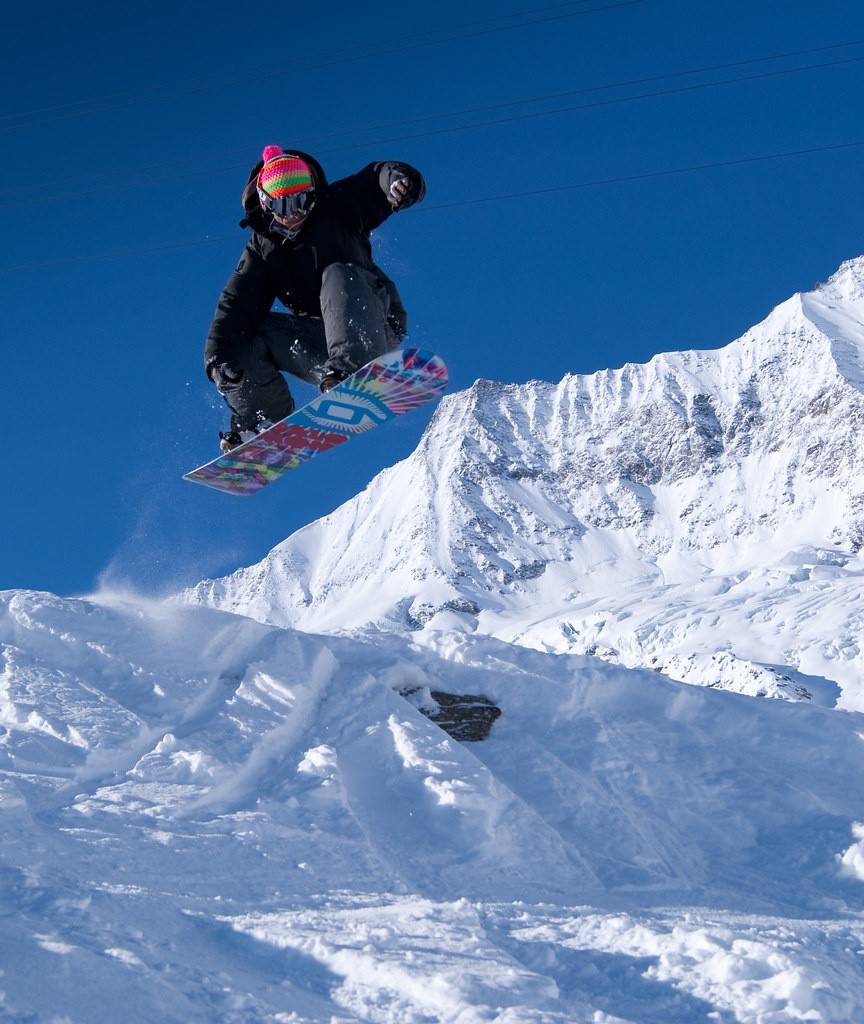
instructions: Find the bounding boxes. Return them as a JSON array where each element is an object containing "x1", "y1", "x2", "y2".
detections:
[{"x1": 259, "y1": 146, "x2": 313, "y2": 199}]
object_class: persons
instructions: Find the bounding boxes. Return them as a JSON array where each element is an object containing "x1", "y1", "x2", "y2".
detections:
[{"x1": 204, "y1": 143, "x2": 427, "y2": 453}]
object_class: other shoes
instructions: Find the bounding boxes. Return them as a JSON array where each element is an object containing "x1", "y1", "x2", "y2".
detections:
[
  {"x1": 220, "y1": 420, "x2": 275, "y2": 450},
  {"x1": 319, "y1": 368, "x2": 349, "y2": 391}
]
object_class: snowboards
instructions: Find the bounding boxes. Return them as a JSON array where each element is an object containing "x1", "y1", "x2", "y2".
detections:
[{"x1": 182, "y1": 347, "x2": 447, "y2": 496}]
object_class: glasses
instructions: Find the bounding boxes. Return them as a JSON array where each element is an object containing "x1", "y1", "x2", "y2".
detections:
[{"x1": 256, "y1": 166, "x2": 316, "y2": 218}]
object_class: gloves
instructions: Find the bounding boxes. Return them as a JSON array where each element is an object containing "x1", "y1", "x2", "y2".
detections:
[
  {"x1": 210, "y1": 361, "x2": 245, "y2": 396},
  {"x1": 385, "y1": 178, "x2": 409, "y2": 208}
]
